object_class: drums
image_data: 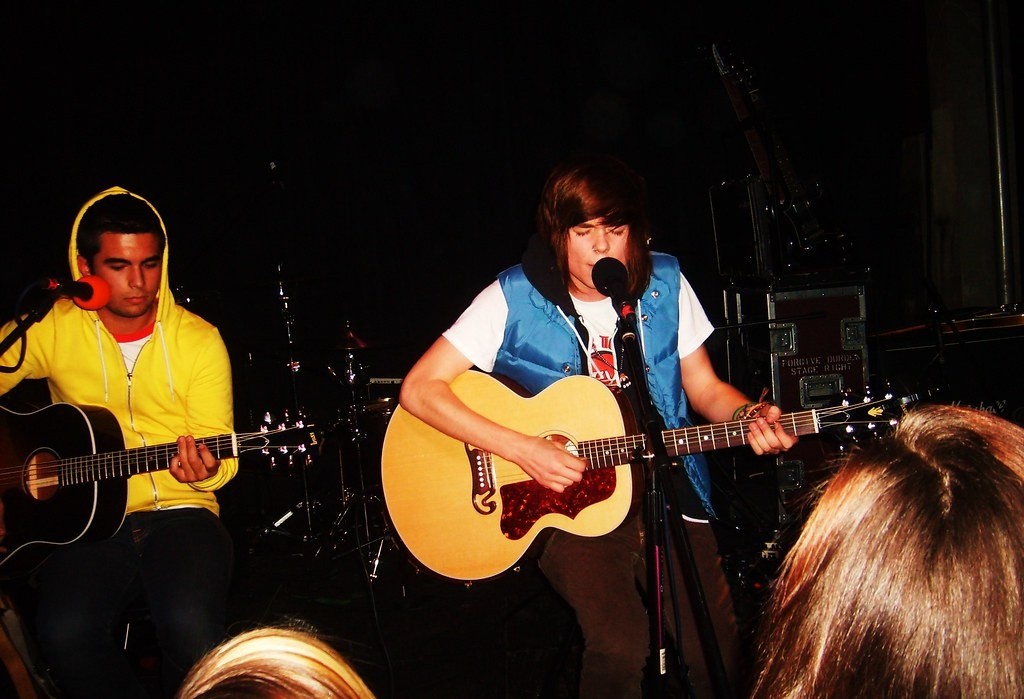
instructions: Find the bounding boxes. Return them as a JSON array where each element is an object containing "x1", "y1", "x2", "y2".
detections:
[
  {"x1": 357, "y1": 376, "x2": 403, "y2": 411},
  {"x1": 360, "y1": 396, "x2": 393, "y2": 424}
]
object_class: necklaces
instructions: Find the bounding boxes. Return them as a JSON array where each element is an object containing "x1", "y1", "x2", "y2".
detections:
[{"x1": 593, "y1": 340, "x2": 632, "y2": 389}]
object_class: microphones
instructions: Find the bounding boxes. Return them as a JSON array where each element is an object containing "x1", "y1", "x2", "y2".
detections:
[
  {"x1": 591, "y1": 257, "x2": 638, "y2": 327},
  {"x1": 26, "y1": 274, "x2": 112, "y2": 312}
]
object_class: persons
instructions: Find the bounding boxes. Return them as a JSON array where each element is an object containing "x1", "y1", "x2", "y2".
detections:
[
  {"x1": 178, "y1": 625, "x2": 378, "y2": 699},
  {"x1": 395, "y1": 151, "x2": 800, "y2": 699},
  {"x1": 749, "y1": 401, "x2": 1023, "y2": 699},
  {"x1": 0, "y1": 185, "x2": 240, "y2": 699}
]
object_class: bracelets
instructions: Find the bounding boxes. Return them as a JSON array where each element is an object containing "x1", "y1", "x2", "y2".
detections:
[{"x1": 732, "y1": 386, "x2": 772, "y2": 422}]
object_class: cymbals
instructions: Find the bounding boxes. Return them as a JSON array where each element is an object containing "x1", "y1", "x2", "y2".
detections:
[{"x1": 324, "y1": 331, "x2": 370, "y2": 351}]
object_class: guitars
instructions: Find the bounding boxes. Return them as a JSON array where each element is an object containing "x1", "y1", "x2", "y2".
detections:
[
  {"x1": 0, "y1": 401, "x2": 325, "y2": 570},
  {"x1": 378, "y1": 369, "x2": 938, "y2": 595}
]
